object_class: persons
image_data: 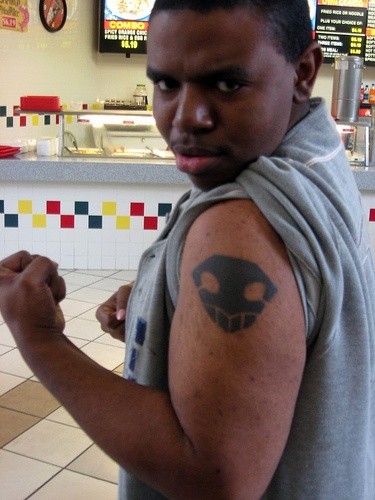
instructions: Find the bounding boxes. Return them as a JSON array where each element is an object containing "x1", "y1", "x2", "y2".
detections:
[{"x1": 0, "y1": 0, "x2": 375, "y2": 500}]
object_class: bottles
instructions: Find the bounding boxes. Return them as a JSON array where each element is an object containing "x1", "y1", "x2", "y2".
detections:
[
  {"x1": 360, "y1": 83, "x2": 375, "y2": 104},
  {"x1": 134, "y1": 85, "x2": 146, "y2": 106}
]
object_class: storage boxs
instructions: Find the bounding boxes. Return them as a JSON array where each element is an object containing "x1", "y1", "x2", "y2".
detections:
[{"x1": 36, "y1": 137, "x2": 58, "y2": 156}]
